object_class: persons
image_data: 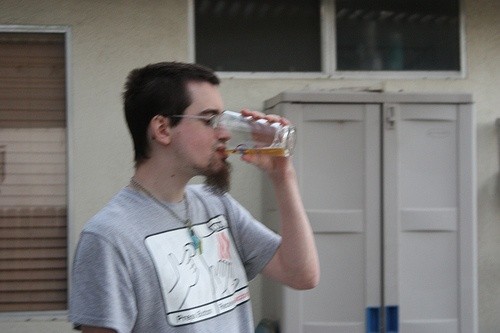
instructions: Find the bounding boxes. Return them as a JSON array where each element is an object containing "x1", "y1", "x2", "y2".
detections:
[{"x1": 66, "y1": 61, "x2": 319, "y2": 333}]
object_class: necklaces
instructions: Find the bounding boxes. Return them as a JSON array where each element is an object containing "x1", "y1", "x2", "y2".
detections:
[{"x1": 131, "y1": 176, "x2": 202, "y2": 246}]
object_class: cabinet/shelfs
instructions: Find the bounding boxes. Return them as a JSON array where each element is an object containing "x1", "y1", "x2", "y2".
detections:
[{"x1": 261, "y1": 89, "x2": 476, "y2": 333}]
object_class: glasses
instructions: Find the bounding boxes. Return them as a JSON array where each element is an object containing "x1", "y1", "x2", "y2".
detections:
[{"x1": 164, "y1": 113, "x2": 216, "y2": 127}]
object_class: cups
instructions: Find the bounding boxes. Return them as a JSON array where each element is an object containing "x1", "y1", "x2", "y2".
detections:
[{"x1": 214, "y1": 110, "x2": 296, "y2": 159}]
